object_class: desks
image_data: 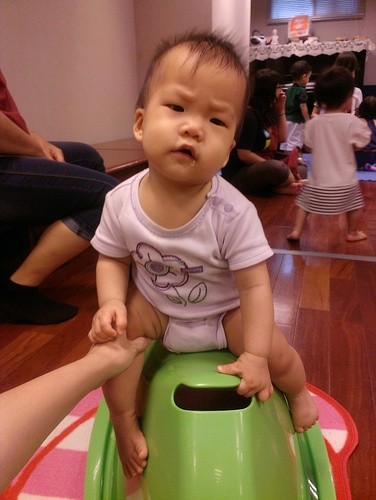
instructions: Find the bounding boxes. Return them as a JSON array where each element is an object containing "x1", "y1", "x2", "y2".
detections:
[{"x1": 250, "y1": 38, "x2": 376, "y2": 94}]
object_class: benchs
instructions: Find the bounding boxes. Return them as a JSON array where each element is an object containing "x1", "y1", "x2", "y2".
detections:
[{"x1": 89, "y1": 138, "x2": 150, "y2": 182}]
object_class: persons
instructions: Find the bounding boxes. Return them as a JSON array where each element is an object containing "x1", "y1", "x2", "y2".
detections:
[
  {"x1": 87, "y1": 35, "x2": 320, "y2": 480},
  {"x1": 0, "y1": 63, "x2": 121, "y2": 326},
  {"x1": 221, "y1": 49, "x2": 376, "y2": 244},
  {"x1": 0, "y1": 316, "x2": 153, "y2": 500}
]
